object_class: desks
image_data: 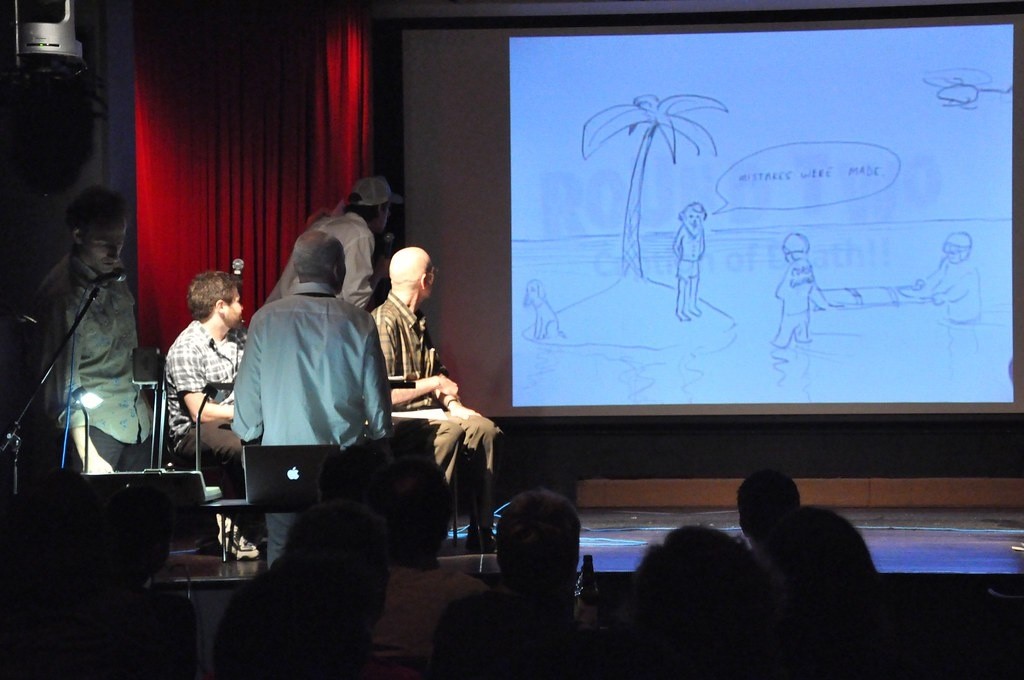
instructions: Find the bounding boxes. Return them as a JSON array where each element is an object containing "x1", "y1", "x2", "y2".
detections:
[{"x1": 200, "y1": 497, "x2": 314, "y2": 513}]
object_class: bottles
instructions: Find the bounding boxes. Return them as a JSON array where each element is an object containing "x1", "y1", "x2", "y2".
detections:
[{"x1": 573, "y1": 555, "x2": 601, "y2": 630}]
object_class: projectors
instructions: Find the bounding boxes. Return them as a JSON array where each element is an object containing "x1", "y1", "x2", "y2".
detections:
[{"x1": 0, "y1": 0, "x2": 87, "y2": 80}]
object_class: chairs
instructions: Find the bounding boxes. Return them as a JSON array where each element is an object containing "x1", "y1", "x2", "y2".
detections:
[{"x1": 132, "y1": 345, "x2": 235, "y2": 563}]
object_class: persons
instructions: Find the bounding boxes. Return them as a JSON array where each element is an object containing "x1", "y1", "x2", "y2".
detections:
[
  {"x1": 210, "y1": 500, "x2": 424, "y2": 680},
  {"x1": 229, "y1": 232, "x2": 395, "y2": 567},
  {"x1": 1, "y1": 466, "x2": 199, "y2": 680},
  {"x1": 30, "y1": 188, "x2": 156, "y2": 492},
  {"x1": 164, "y1": 272, "x2": 260, "y2": 563},
  {"x1": 430, "y1": 465, "x2": 938, "y2": 679},
  {"x1": 263, "y1": 176, "x2": 402, "y2": 307},
  {"x1": 372, "y1": 246, "x2": 503, "y2": 552}
]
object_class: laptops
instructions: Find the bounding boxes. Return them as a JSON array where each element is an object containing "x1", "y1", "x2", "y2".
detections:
[{"x1": 243, "y1": 445, "x2": 341, "y2": 507}]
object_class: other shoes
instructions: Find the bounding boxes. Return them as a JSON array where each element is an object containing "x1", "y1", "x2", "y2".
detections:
[
  {"x1": 218, "y1": 525, "x2": 259, "y2": 559},
  {"x1": 467, "y1": 525, "x2": 496, "y2": 551}
]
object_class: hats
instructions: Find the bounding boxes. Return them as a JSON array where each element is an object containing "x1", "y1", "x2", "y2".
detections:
[{"x1": 350, "y1": 176, "x2": 403, "y2": 206}]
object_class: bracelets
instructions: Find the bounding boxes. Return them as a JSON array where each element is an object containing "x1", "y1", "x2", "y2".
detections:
[{"x1": 448, "y1": 399, "x2": 459, "y2": 407}]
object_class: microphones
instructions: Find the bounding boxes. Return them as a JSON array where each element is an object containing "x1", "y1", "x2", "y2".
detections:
[
  {"x1": 383, "y1": 232, "x2": 395, "y2": 259},
  {"x1": 93, "y1": 267, "x2": 127, "y2": 284},
  {"x1": 232, "y1": 259, "x2": 244, "y2": 280}
]
